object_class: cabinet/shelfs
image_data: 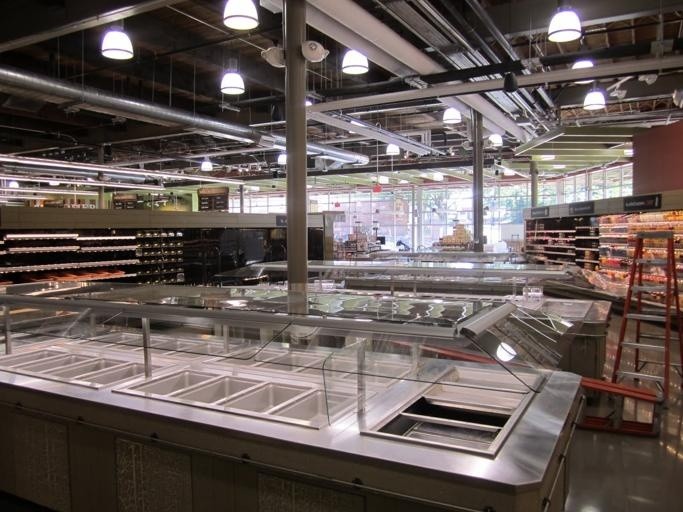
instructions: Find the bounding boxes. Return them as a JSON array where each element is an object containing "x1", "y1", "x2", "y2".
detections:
[
  {"x1": 2, "y1": 206, "x2": 334, "y2": 297},
  {"x1": 525, "y1": 192, "x2": 682, "y2": 325}
]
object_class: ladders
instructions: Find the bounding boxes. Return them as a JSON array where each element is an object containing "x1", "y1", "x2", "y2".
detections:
[
  {"x1": 611, "y1": 230, "x2": 679, "y2": 409},
  {"x1": 374, "y1": 338, "x2": 662, "y2": 437}
]
